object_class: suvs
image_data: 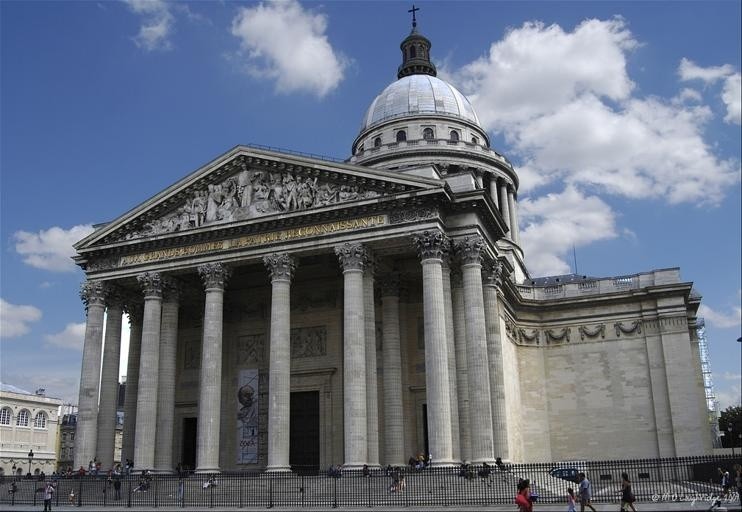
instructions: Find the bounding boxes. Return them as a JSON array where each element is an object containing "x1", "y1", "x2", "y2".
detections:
[{"x1": 551, "y1": 468, "x2": 585, "y2": 484}]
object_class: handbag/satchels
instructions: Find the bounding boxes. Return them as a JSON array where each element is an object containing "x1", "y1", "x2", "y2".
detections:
[{"x1": 516, "y1": 495, "x2": 530, "y2": 508}]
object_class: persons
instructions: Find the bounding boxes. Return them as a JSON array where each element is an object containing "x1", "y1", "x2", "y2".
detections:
[
  {"x1": 717, "y1": 464, "x2": 732, "y2": 499},
  {"x1": 622, "y1": 484, "x2": 630, "y2": 511},
  {"x1": 336, "y1": 464, "x2": 341, "y2": 477},
  {"x1": 112, "y1": 477, "x2": 122, "y2": 501},
  {"x1": 386, "y1": 448, "x2": 507, "y2": 493},
  {"x1": 363, "y1": 463, "x2": 372, "y2": 477},
  {"x1": 614, "y1": 471, "x2": 638, "y2": 511},
  {"x1": 46, "y1": 456, "x2": 218, "y2": 492},
  {"x1": 579, "y1": 471, "x2": 597, "y2": 512},
  {"x1": 237, "y1": 384, "x2": 259, "y2": 430},
  {"x1": 328, "y1": 463, "x2": 339, "y2": 477},
  {"x1": 566, "y1": 486, "x2": 578, "y2": 512},
  {"x1": 515, "y1": 478, "x2": 538, "y2": 511},
  {"x1": 364, "y1": 465, "x2": 370, "y2": 474},
  {"x1": 44, "y1": 481, "x2": 56, "y2": 512}
]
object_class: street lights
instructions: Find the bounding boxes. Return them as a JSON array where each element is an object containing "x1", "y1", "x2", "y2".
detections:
[
  {"x1": 12, "y1": 463, "x2": 17, "y2": 476},
  {"x1": 728, "y1": 423, "x2": 737, "y2": 461},
  {"x1": 24, "y1": 449, "x2": 34, "y2": 480}
]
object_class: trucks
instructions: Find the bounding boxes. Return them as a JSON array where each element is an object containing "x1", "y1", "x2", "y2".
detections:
[{"x1": 549, "y1": 460, "x2": 589, "y2": 480}]
